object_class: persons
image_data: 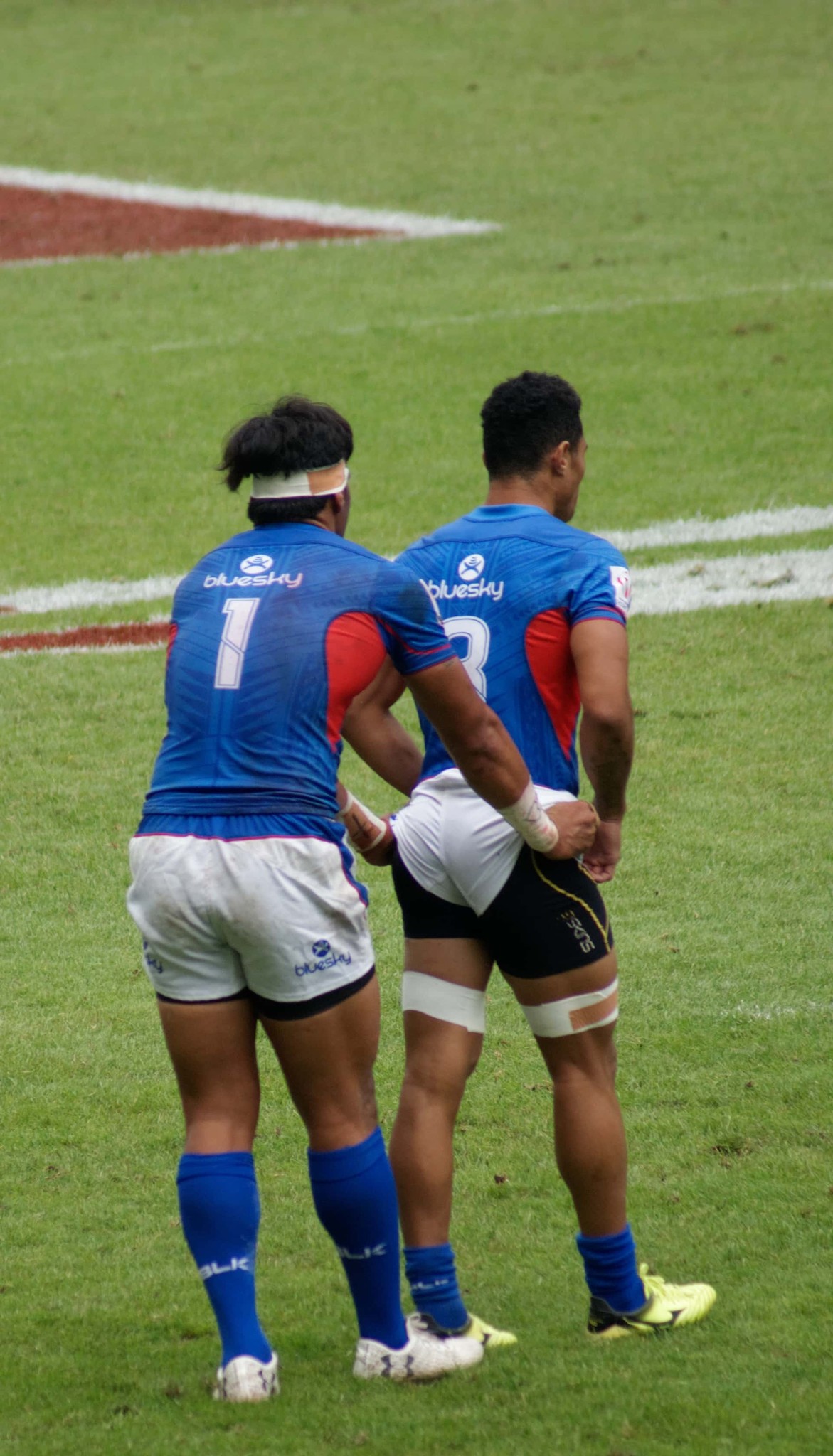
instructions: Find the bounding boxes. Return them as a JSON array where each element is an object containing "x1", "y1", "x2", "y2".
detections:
[
  {"x1": 339, "y1": 369, "x2": 717, "y2": 1351},
  {"x1": 128, "y1": 400, "x2": 603, "y2": 1400}
]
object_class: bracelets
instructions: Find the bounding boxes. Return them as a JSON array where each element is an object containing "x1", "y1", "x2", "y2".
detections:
[
  {"x1": 339, "y1": 791, "x2": 387, "y2": 853},
  {"x1": 497, "y1": 775, "x2": 559, "y2": 853}
]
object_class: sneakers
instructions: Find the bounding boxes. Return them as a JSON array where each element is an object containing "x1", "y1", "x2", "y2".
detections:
[
  {"x1": 353, "y1": 1313, "x2": 484, "y2": 1381},
  {"x1": 212, "y1": 1352, "x2": 280, "y2": 1403},
  {"x1": 586, "y1": 1262, "x2": 716, "y2": 1341},
  {"x1": 422, "y1": 1311, "x2": 517, "y2": 1347}
]
